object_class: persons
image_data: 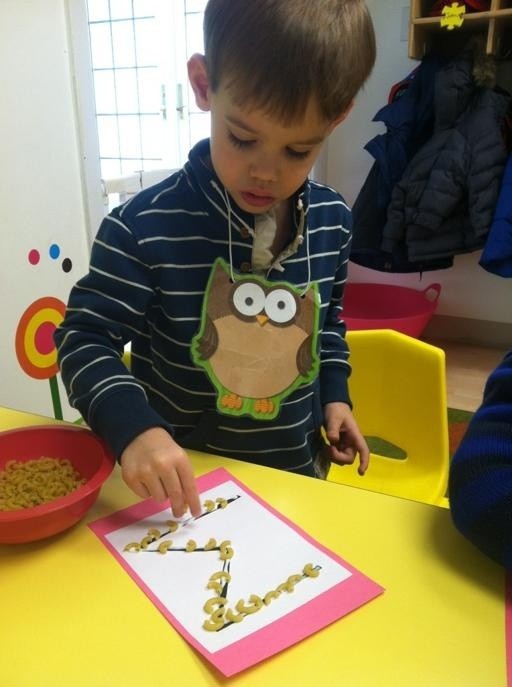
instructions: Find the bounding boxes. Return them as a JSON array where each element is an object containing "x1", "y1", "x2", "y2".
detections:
[
  {"x1": 52, "y1": 0, "x2": 377, "y2": 519},
  {"x1": 446, "y1": 347, "x2": 512, "y2": 573}
]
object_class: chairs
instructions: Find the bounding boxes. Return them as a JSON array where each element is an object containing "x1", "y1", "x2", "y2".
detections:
[{"x1": 327, "y1": 329, "x2": 449, "y2": 503}]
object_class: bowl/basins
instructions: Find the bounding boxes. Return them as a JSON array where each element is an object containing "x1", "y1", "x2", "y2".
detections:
[
  {"x1": 337, "y1": 279, "x2": 444, "y2": 341},
  {"x1": 1, "y1": 425, "x2": 117, "y2": 543}
]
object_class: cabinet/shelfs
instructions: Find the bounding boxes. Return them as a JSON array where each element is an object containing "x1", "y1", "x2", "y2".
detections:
[{"x1": 408, "y1": 0, "x2": 512, "y2": 59}]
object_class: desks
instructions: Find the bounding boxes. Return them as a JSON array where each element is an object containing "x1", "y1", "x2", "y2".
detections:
[{"x1": 1, "y1": 409, "x2": 511, "y2": 687}]
